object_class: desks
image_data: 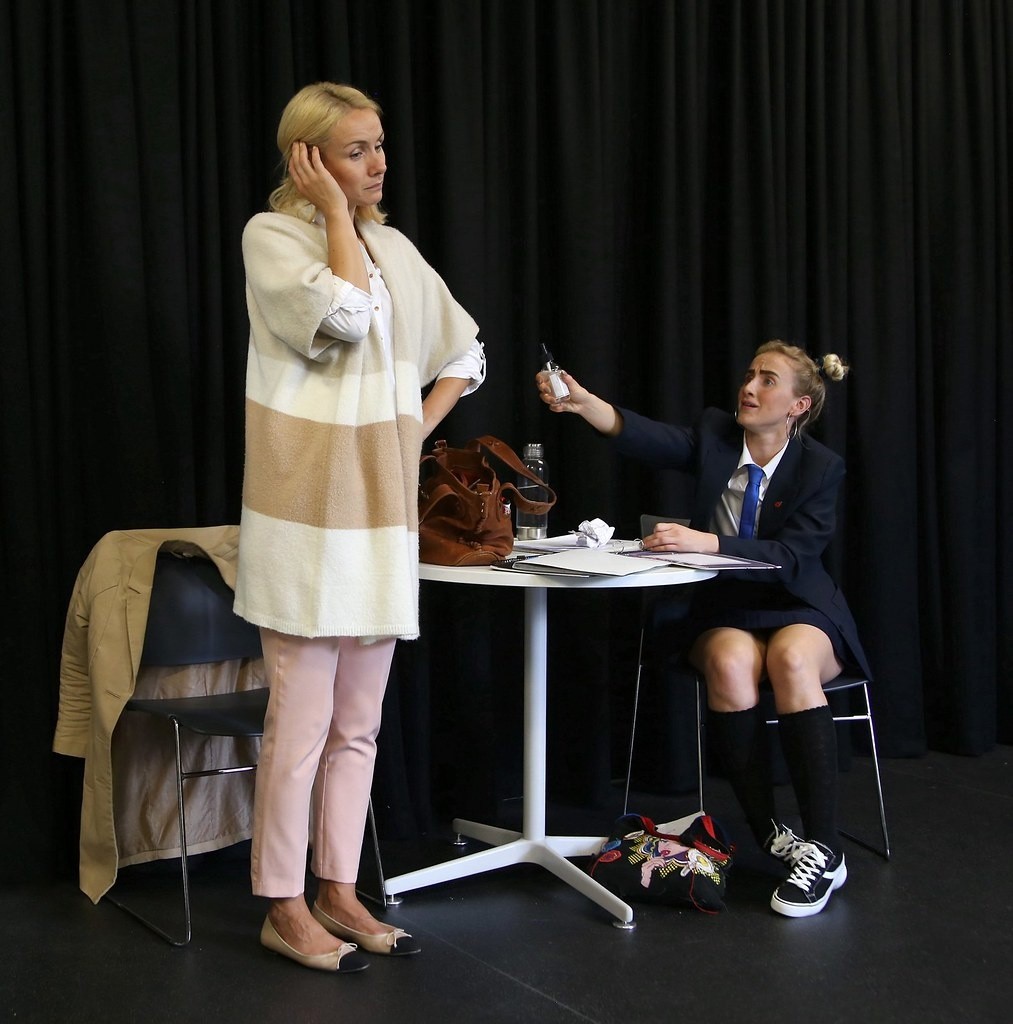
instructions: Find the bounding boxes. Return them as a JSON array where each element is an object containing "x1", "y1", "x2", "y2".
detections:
[{"x1": 384, "y1": 537, "x2": 717, "y2": 931}]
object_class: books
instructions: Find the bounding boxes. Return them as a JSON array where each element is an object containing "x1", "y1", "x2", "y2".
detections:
[{"x1": 489, "y1": 556, "x2": 593, "y2": 578}]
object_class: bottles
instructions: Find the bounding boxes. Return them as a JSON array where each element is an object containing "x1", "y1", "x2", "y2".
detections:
[
  {"x1": 516, "y1": 444, "x2": 548, "y2": 541},
  {"x1": 539, "y1": 342, "x2": 571, "y2": 403}
]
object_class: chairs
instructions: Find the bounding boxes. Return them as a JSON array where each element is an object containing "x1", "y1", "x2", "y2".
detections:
[
  {"x1": 621, "y1": 515, "x2": 890, "y2": 864},
  {"x1": 50, "y1": 525, "x2": 386, "y2": 947}
]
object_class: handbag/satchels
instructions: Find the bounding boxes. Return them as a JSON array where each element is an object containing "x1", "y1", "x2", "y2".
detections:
[
  {"x1": 590, "y1": 813, "x2": 737, "y2": 914},
  {"x1": 418, "y1": 436, "x2": 557, "y2": 567}
]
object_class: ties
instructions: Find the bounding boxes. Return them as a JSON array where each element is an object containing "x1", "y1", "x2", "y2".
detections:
[{"x1": 738, "y1": 464, "x2": 764, "y2": 540}]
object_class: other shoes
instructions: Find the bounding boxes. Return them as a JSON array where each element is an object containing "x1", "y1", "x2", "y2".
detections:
[
  {"x1": 261, "y1": 914, "x2": 369, "y2": 973},
  {"x1": 311, "y1": 900, "x2": 422, "y2": 955}
]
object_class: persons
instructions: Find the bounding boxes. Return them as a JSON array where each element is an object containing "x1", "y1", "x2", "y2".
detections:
[
  {"x1": 537, "y1": 342, "x2": 871, "y2": 918},
  {"x1": 233, "y1": 81, "x2": 485, "y2": 973}
]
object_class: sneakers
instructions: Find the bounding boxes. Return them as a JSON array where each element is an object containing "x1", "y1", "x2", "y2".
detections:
[
  {"x1": 763, "y1": 819, "x2": 806, "y2": 871},
  {"x1": 771, "y1": 839, "x2": 847, "y2": 918}
]
